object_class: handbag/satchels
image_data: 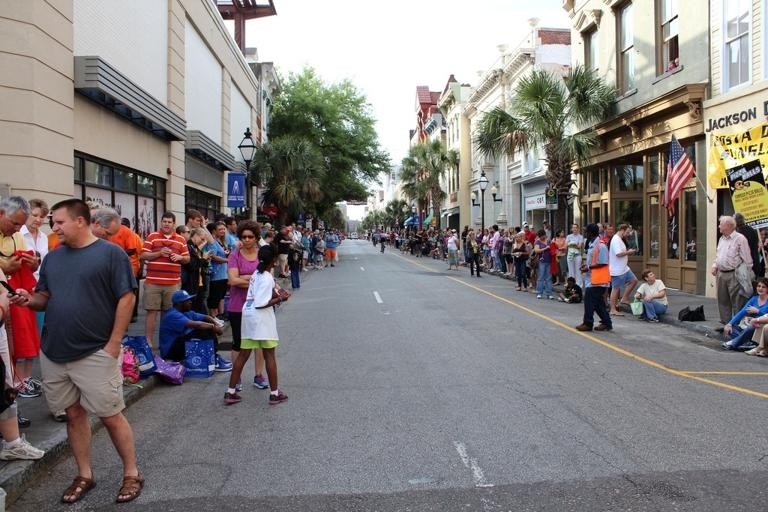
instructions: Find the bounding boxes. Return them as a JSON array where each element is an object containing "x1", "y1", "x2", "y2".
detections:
[
  {"x1": 527, "y1": 249, "x2": 541, "y2": 270},
  {"x1": 737, "y1": 315, "x2": 756, "y2": 331},
  {"x1": 121, "y1": 333, "x2": 218, "y2": 387},
  {"x1": 629, "y1": 293, "x2": 644, "y2": 316}
]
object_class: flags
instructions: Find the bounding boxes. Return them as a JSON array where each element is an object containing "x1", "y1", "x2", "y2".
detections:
[{"x1": 661, "y1": 134, "x2": 695, "y2": 217}]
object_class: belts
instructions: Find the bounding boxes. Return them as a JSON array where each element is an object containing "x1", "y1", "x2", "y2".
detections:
[{"x1": 718, "y1": 261, "x2": 743, "y2": 273}]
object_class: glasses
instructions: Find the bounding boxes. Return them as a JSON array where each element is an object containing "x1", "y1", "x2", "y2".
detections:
[
  {"x1": 182, "y1": 231, "x2": 191, "y2": 234},
  {"x1": 241, "y1": 234, "x2": 256, "y2": 239}
]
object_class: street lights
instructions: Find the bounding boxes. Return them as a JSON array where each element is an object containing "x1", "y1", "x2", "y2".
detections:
[
  {"x1": 411, "y1": 206, "x2": 417, "y2": 229},
  {"x1": 477, "y1": 171, "x2": 488, "y2": 236},
  {"x1": 238, "y1": 127, "x2": 257, "y2": 218}
]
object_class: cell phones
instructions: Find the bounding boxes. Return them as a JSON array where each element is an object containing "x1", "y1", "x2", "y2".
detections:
[{"x1": 0, "y1": 281, "x2": 28, "y2": 303}]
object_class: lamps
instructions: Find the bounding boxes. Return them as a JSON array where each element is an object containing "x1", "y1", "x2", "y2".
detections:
[
  {"x1": 490, "y1": 186, "x2": 502, "y2": 202},
  {"x1": 470, "y1": 193, "x2": 481, "y2": 207}
]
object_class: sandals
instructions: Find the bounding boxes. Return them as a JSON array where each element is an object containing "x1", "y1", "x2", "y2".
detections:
[
  {"x1": 61, "y1": 469, "x2": 97, "y2": 504},
  {"x1": 116, "y1": 471, "x2": 145, "y2": 503}
]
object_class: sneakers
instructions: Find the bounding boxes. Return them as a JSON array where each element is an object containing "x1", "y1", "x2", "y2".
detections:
[
  {"x1": 594, "y1": 323, "x2": 614, "y2": 331},
  {"x1": 253, "y1": 375, "x2": 270, "y2": 389},
  {"x1": 610, "y1": 310, "x2": 626, "y2": 317},
  {"x1": 215, "y1": 354, "x2": 234, "y2": 372},
  {"x1": 575, "y1": 323, "x2": 594, "y2": 331},
  {"x1": 277, "y1": 264, "x2": 336, "y2": 279},
  {"x1": 721, "y1": 341, "x2": 757, "y2": 350},
  {"x1": 18, "y1": 412, "x2": 32, "y2": 428},
  {"x1": 235, "y1": 377, "x2": 243, "y2": 391},
  {"x1": 269, "y1": 388, "x2": 288, "y2": 404},
  {"x1": 223, "y1": 392, "x2": 242, "y2": 404},
  {"x1": 745, "y1": 348, "x2": 768, "y2": 356},
  {"x1": 13, "y1": 376, "x2": 42, "y2": 398},
  {"x1": 0, "y1": 431, "x2": 48, "y2": 460},
  {"x1": 447, "y1": 263, "x2": 571, "y2": 304},
  {"x1": 638, "y1": 316, "x2": 660, "y2": 323}
]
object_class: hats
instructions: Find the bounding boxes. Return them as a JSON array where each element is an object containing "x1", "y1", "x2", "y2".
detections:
[
  {"x1": 522, "y1": 219, "x2": 548, "y2": 230},
  {"x1": 172, "y1": 291, "x2": 197, "y2": 302}
]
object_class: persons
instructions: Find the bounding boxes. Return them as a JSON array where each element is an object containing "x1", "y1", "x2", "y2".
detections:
[
  {"x1": 733, "y1": 213, "x2": 758, "y2": 279},
  {"x1": 373, "y1": 220, "x2": 638, "y2": 331},
  {"x1": 744, "y1": 314, "x2": 768, "y2": 357},
  {"x1": 635, "y1": 269, "x2": 668, "y2": 322},
  {"x1": 1, "y1": 195, "x2": 339, "y2": 458},
  {"x1": 720, "y1": 277, "x2": 768, "y2": 350},
  {"x1": 712, "y1": 215, "x2": 754, "y2": 331},
  {"x1": 757, "y1": 228, "x2": 768, "y2": 277},
  {"x1": 7, "y1": 199, "x2": 144, "y2": 502}
]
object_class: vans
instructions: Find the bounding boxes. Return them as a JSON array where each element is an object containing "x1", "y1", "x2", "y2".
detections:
[{"x1": 350, "y1": 231, "x2": 359, "y2": 239}]
